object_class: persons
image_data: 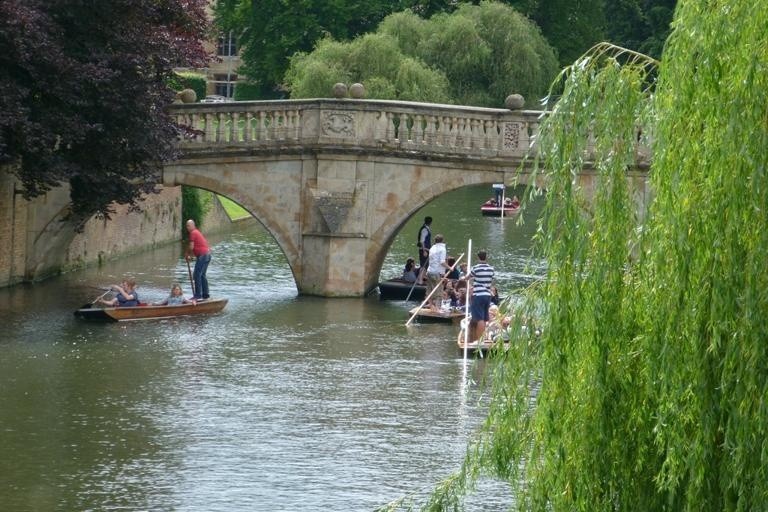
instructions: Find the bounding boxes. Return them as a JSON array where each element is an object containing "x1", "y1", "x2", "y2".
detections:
[
  {"x1": 185, "y1": 218, "x2": 211, "y2": 302},
  {"x1": 400, "y1": 213, "x2": 536, "y2": 346},
  {"x1": 97, "y1": 280, "x2": 138, "y2": 307},
  {"x1": 490, "y1": 183, "x2": 520, "y2": 208},
  {"x1": 148, "y1": 283, "x2": 196, "y2": 307}
]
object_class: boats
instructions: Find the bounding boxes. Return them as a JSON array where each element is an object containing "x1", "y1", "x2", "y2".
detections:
[
  {"x1": 409, "y1": 297, "x2": 511, "y2": 324},
  {"x1": 74, "y1": 298, "x2": 228, "y2": 322},
  {"x1": 456, "y1": 315, "x2": 543, "y2": 351},
  {"x1": 378, "y1": 266, "x2": 471, "y2": 297},
  {"x1": 480, "y1": 198, "x2": 520, "y2": 214}
]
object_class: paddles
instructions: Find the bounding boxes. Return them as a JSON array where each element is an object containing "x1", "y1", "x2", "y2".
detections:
[{"x1": 80, "y1": 287, "x2": 113, "y2": 309}]
